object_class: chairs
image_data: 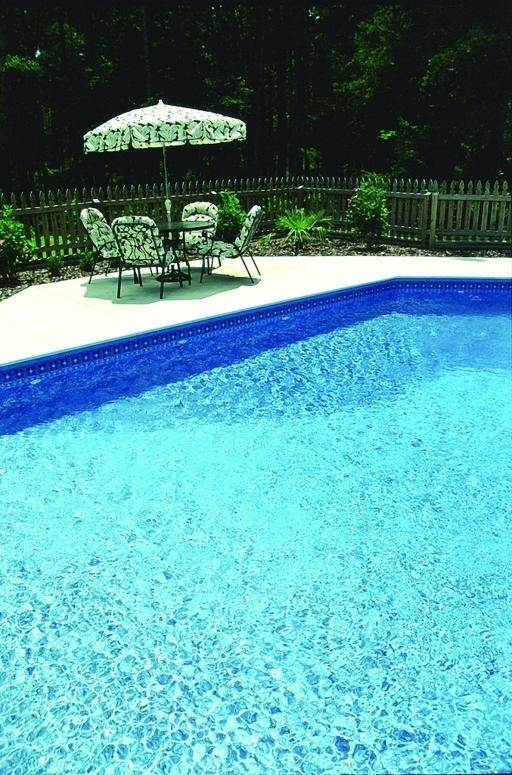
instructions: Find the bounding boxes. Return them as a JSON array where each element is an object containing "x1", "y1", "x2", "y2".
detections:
[{"x1": 80, "y1": 200, "x2": 263, "y2": 300}]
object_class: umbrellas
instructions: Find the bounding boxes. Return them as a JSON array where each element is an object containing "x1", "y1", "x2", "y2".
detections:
[{"x1": 83, "y1": 99, "x2": 246, "y2": 253}]
